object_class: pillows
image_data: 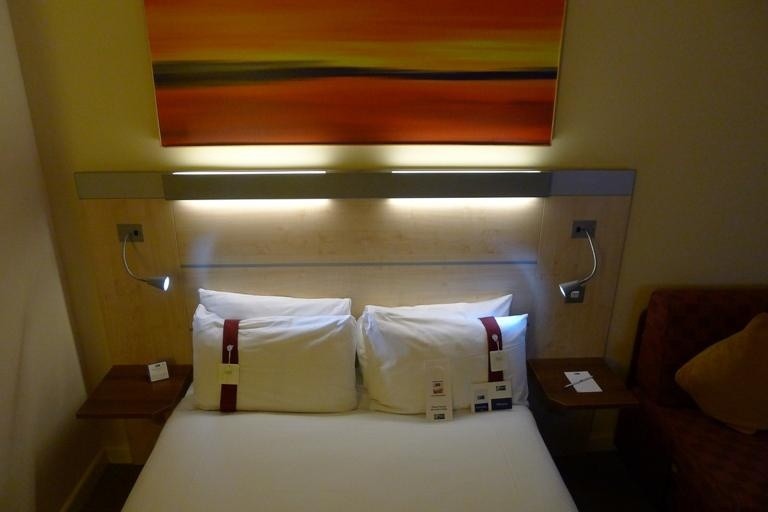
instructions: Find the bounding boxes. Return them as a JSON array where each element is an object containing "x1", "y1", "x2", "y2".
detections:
[
  {"x1": 365, "y1": 294, "x2": 513, "y2": 318},
  {"x1": 193, "y1": 303, "x2": 357, "y2": 413},
  {"x1": 675, "y1": 313, "x2": 768, "y2": 435},
  {"x1": 197, "y1": 288, "x2": 351, "y2": 321},
  {"x1": 357, "y1": 309, "x2": 529, "y2": 414}
]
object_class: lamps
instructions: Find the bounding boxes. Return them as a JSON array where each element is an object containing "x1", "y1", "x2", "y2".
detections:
[
  {"x1": 117, "y1": 224, "x2": 170, "y2": 292},
  {"x1": 559, "y1": 220, "x2": 598, "y2": 297}
]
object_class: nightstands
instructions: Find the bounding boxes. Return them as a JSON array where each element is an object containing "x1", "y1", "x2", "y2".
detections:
[
  {"x1": 528, "y1": 357, "x2": 640, "y2": 411},
  {"x1": 75, "y1": 364, "x2": 193, "y2": 427}
]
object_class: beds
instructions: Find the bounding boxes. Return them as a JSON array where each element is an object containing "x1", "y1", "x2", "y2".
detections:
[{"x1": 74, "y1": 167, "x2": 634, "y2": 512}]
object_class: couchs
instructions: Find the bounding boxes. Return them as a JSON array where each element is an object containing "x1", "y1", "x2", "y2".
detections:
[{"x1": 617, "y1": 287, "x2": 768, "y2": 512}]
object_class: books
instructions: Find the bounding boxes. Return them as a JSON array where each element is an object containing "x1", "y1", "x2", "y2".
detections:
[
  {"x1": 423, "y1": 376, "x2": 454, "y2": 422},
  {"x1": 564, "y1": 371, "x2": 603, "y2": 393},
  {"x1": 470, "y1": 380, "x2": 513, "y2": 413}
]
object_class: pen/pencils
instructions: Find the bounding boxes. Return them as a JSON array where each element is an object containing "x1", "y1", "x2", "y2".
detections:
[{"x1": 564, "y1": 376, "x2": 593, "y2": 388}]
